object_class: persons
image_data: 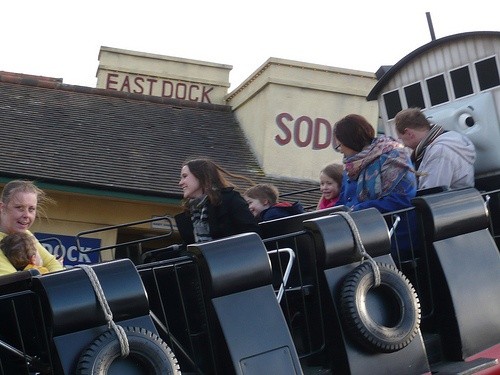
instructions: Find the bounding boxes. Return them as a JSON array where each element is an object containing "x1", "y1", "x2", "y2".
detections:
[
  {"x1": 0, "y1": 180, "x2": 65, "y2": 276},
  {"x1": 314, "y1": 108, "x2": 477, "y2": 212},
  {"x1": 175, "y1": 158, "x2": 305, "y2": 247}
]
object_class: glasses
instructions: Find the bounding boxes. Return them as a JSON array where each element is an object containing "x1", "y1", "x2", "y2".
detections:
[{"x1": 334, "y1": 142, "x2": 342, "y2": 152}]
